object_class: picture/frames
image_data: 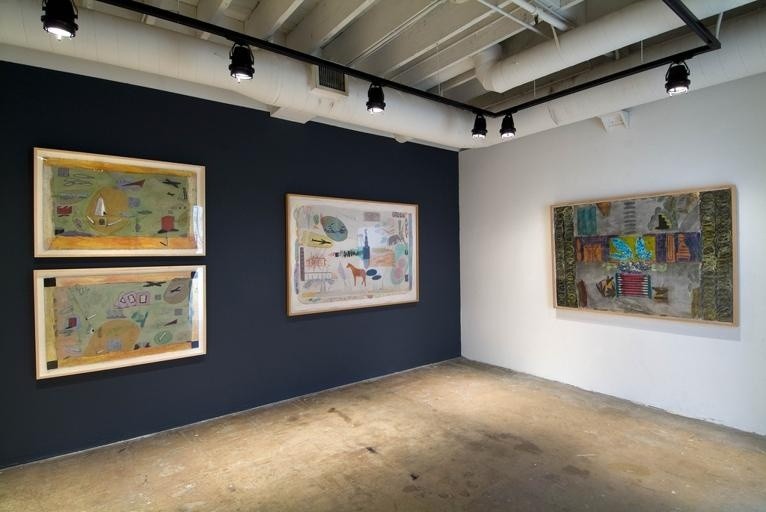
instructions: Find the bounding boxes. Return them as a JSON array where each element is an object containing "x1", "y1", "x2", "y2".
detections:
[
  {"x1": 282, "y1": 190, "x2": 422, "y2": 320},
  {"x1": 546, "y1": 183, "x2": 739, "y2": 329},
  {"x1": 28, "y1": 262, "x2": 208, "y2": 383},
  {"x1": 31, "y1": 143, "x2": 206, "y2": 260}
]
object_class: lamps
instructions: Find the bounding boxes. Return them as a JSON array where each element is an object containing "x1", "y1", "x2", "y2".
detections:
[
  {"x1": 221, "y1": 33, "x2": 262, "y2": 84},
  {"x1": 665, "y1": 57, "x2": 694, "y2": 96},
  {"x1": 470, "y1": 110, "x2": 484, "y2": 138},
  {"x1": 35, "y1": 0, "x2": 79, "y2": 42},
  {"x1": 497, "y1": 108, "x2": 519, "y2": 141},
  {"x1": 363, "y1": 74, "x2": 384, "y2": 112}
]
